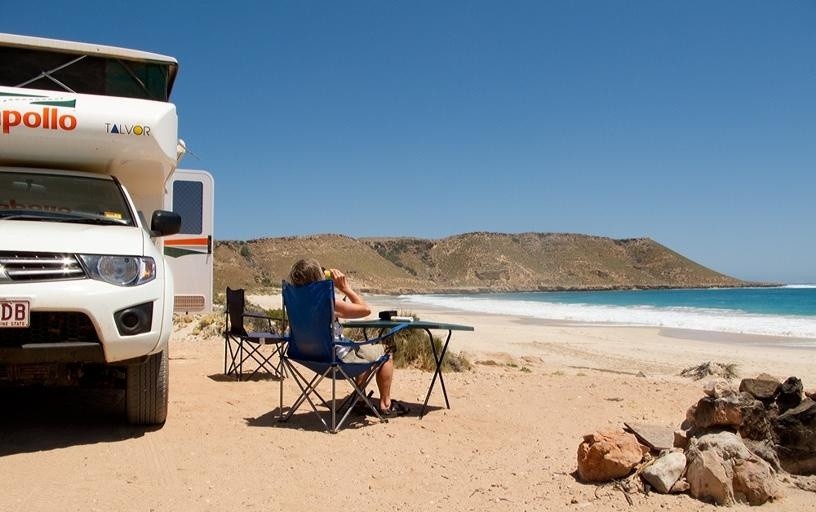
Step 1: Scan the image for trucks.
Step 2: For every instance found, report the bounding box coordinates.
[1,27,225,440]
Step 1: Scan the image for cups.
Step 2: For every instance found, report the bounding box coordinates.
[324,270,330,279]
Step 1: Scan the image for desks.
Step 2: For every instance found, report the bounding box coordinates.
[338,319,476,420]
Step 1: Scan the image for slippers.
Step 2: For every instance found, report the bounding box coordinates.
[353,400,379,414]
[378,400,411,418]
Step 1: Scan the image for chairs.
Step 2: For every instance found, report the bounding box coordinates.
[223,287,290,380]
[276,283,390,432]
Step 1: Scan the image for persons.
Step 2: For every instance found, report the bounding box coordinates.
[287,257,411,419]
